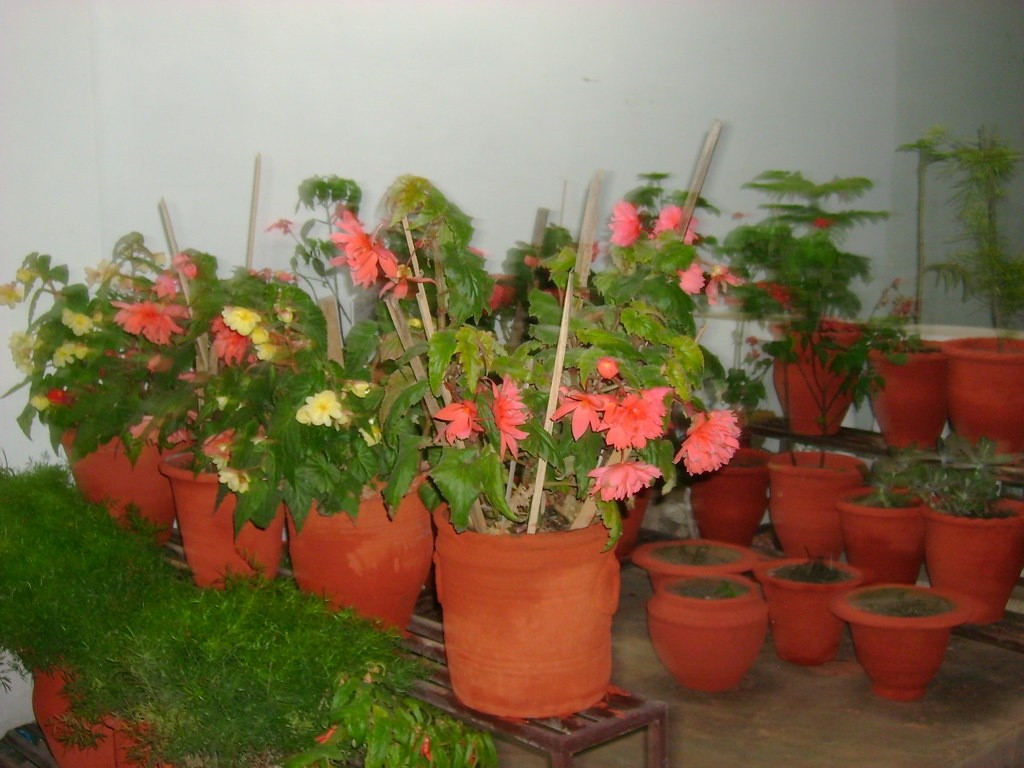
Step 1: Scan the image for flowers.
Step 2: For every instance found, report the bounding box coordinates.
[281,664,499,768]
[0,161,944,554]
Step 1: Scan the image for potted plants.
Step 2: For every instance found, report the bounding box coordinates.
[719,170,891,437]
[863,135,947,452]
[0,463,437,768]
[898,130,1024,464]
[835,444,935,586]
[633,535,958,701]
[913,433,1024,626]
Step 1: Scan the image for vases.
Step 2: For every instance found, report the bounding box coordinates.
[58,420,179,544]
[287,471,433,641]
[689,447,775,545]
[434,501,620,719]
[615,483,656,557]
[160,453,287,588]
[768,453,868,556]
